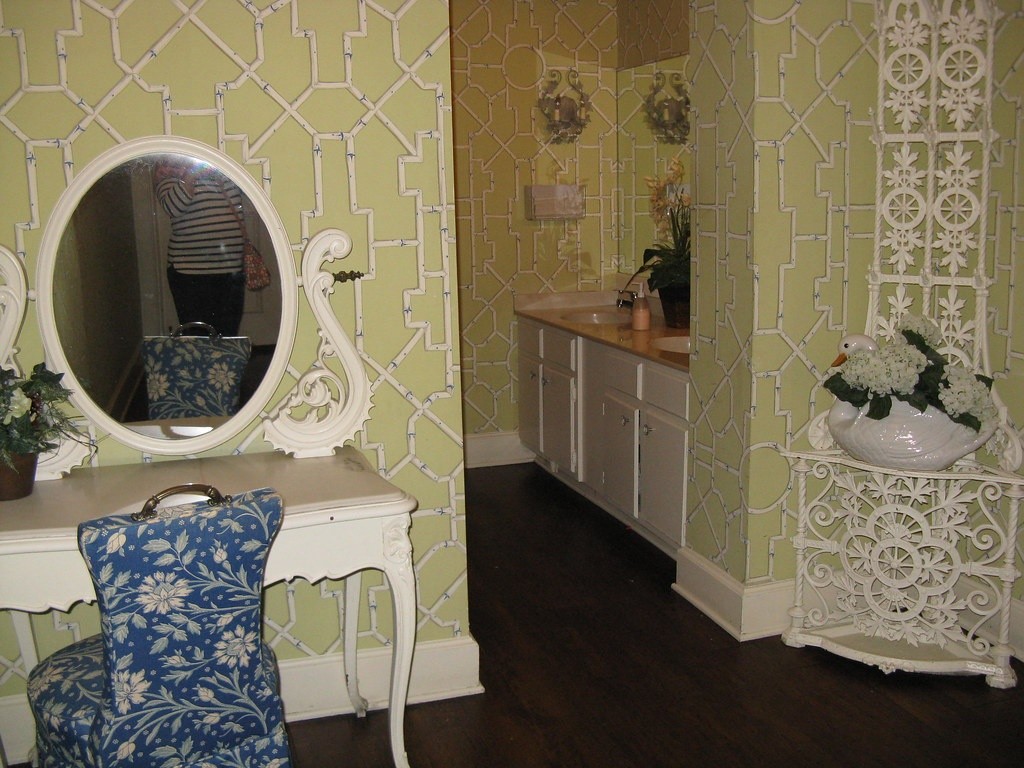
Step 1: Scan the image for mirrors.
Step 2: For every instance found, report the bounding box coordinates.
[37,136,299,456]
[617,53,696,277]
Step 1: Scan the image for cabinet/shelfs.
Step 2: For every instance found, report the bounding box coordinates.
[514,305,691,561]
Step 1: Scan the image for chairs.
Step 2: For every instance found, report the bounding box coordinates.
[142,322,250,418]
[27,483,291,768]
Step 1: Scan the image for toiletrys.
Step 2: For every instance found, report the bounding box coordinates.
[631,281,650,331]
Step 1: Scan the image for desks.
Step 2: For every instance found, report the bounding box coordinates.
[0,444,419,768]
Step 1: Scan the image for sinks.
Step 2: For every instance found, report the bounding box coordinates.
[649,336,690,354]
[558,311,633,326]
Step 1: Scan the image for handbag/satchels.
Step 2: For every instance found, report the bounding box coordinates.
[243,242,271,291]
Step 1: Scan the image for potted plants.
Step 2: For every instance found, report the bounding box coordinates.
[0,362,98,501]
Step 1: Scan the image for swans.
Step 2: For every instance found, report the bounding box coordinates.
[827,333,999,473]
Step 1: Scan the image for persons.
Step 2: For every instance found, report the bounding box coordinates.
[153,156,247,338]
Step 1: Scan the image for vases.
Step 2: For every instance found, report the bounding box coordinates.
[658,285,691,329]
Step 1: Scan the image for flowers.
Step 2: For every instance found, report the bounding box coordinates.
[616,159,690,294]
[824,311,1000,433]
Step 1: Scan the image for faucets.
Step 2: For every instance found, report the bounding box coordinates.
[612,289,637,308]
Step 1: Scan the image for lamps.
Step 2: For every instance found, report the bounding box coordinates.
[643,71,691,144]
[540,67,593,143]
